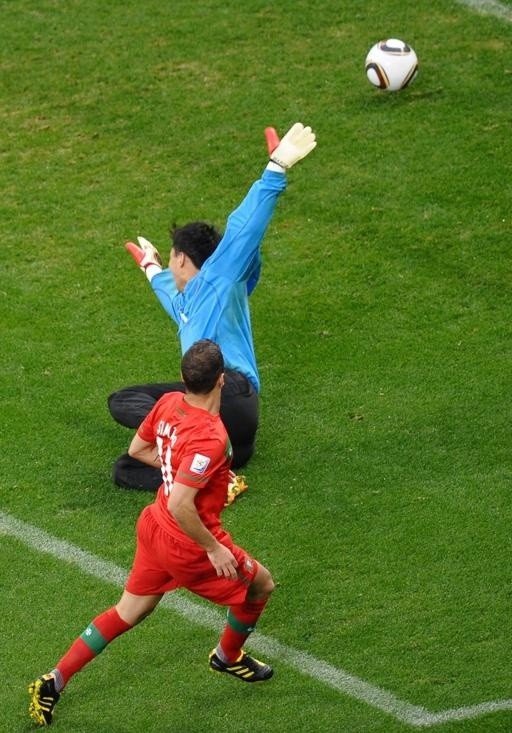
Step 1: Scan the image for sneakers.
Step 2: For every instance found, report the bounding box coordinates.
[27,672,60,728]
[208,647,274,683]
[222,469,249,508]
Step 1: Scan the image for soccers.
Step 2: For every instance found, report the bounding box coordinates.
[364,38,420,95]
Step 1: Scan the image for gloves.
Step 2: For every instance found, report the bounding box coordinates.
[263,123,318,173]
[124,236,163,282]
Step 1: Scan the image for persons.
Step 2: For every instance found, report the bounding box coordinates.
[25,335,279,728]
[102,118,321,511]
